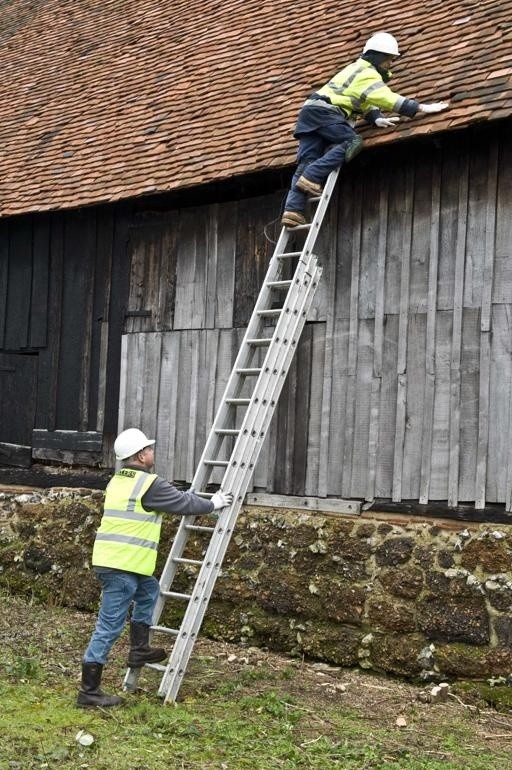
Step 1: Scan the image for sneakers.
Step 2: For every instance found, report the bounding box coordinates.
[295,175,323,197]
[281,211,309,237]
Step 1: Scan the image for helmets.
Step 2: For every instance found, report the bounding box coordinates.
[362,31,401,57]
[113,427,156,462]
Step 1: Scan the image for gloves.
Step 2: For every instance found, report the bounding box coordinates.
[375,116,400,128]
[420,100,449,114]
[209,488,235,510]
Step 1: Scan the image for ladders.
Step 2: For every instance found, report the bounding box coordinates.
[122,153,341,706]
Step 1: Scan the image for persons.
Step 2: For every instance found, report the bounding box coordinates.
[280,31,450,230]
[71,427,234,711]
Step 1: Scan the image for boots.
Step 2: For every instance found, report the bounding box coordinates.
[127,619,168,668]
[75,661,126,709]
